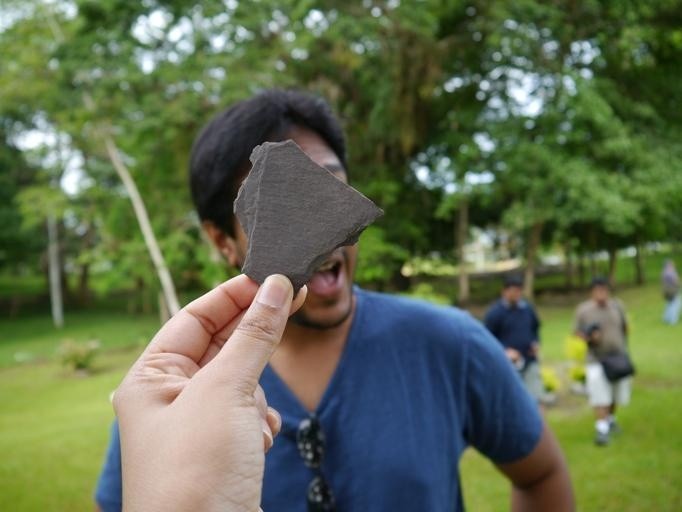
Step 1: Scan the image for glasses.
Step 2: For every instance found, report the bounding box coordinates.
[283,412,336,511]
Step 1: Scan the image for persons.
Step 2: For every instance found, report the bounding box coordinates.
[480,272,545,409]
[568,276,633,447]
[93,86,577,511]
[658,257,681,326]
[111,271,310,511]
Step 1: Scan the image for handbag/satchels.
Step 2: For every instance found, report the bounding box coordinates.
[601,350,637,382]
